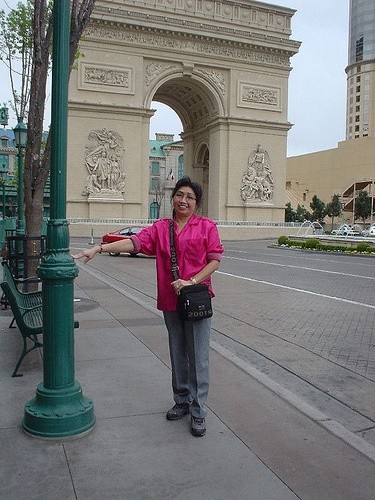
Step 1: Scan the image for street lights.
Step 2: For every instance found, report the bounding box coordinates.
[12,117,28,277]
[0,163,9,219]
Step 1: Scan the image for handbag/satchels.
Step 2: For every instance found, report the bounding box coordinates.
[177,284,213,321]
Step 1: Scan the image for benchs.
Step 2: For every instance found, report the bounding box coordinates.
[0,257,79,377]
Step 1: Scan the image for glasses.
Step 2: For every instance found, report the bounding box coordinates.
[175,193,197,201]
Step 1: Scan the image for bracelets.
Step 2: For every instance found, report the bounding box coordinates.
[190,276,197,285]
[99,244,103,254]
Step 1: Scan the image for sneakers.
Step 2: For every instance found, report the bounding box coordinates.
[190,415,206,436]
[166,403,190,420]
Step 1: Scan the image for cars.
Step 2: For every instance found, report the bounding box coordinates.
[330,225,359,235]
[100,225,148,257]
[361,225,375,238]
[312,223,325,234]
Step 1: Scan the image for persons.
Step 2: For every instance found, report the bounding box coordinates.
[72,177,224,435]
[86,132,119,158]
[86,151,121,189]
[243,145,273,198]
